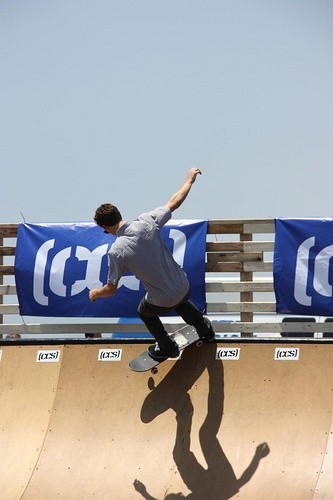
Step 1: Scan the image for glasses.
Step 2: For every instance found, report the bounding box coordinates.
[103,230,109,234]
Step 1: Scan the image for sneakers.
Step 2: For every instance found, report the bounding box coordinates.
[203,317,216,341]
[146,344,179,358]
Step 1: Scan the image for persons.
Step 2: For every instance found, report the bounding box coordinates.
[87,167,215,359]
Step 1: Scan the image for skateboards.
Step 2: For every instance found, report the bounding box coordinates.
[128,317,211,374]
